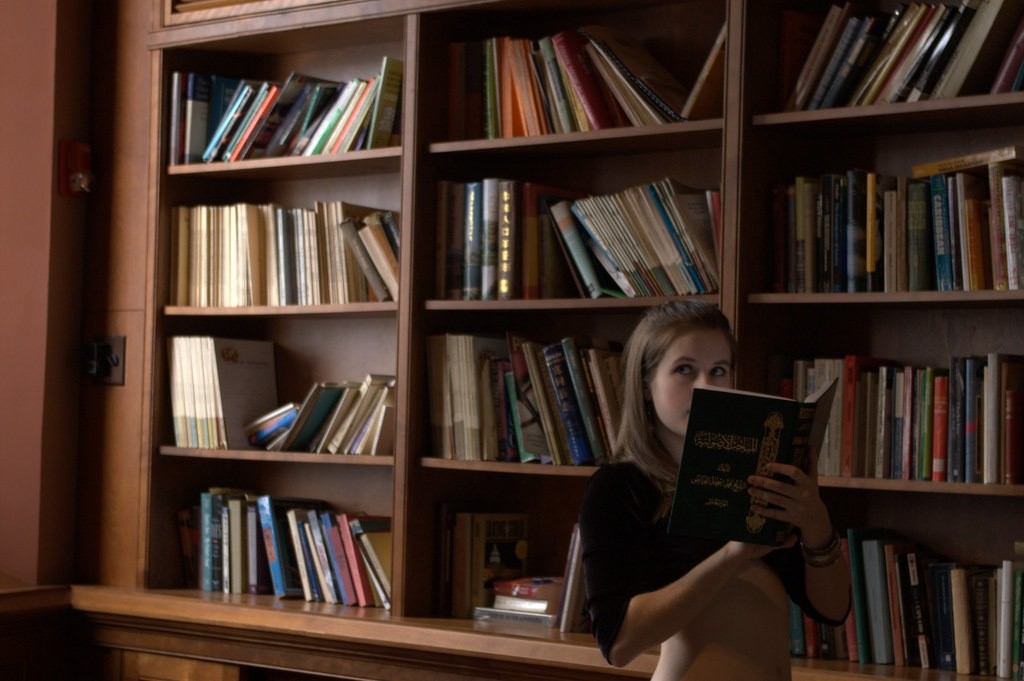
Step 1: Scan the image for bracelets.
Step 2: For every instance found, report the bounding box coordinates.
[801,528,844,569]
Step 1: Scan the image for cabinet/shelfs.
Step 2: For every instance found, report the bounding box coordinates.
[133,0,1024,681]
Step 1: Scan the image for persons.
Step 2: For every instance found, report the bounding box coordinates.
[578,298,853,681]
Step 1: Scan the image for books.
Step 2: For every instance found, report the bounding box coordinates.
[779,351,1024,484]
[481,21,727,141]
[430,336,631,466]
[784,1,1024,109]
[174,332,395,463]
[786,147,1024,294]
[169,56,405,165]
[174,198,407,307]
[174,475,390,612]
[784,525,1023,680]
[437,177,724,299]
[664,375,840,546]
[436,504,590,640]
